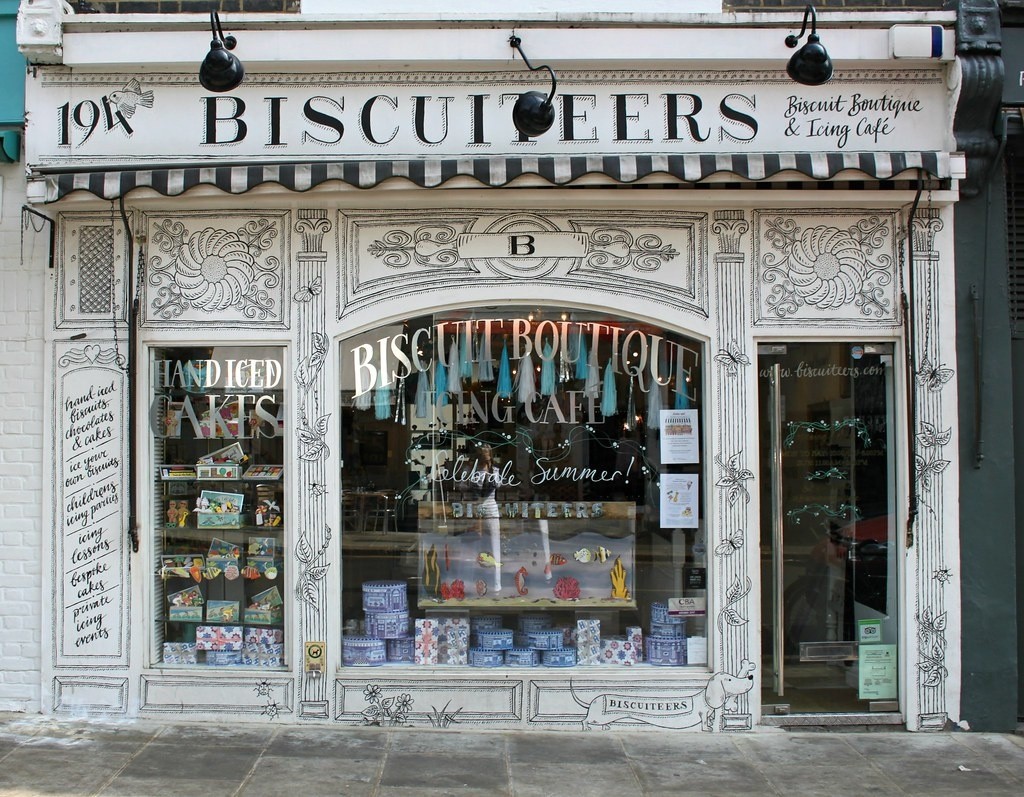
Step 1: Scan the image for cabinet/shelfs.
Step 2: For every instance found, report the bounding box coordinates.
[153,478,286,629]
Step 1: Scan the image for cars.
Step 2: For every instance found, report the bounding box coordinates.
[807,515,888,611]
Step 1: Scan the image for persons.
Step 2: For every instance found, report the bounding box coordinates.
[511,437,555,582]
[454,441,503,597]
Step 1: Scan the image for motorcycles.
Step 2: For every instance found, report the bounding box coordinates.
[784,530,887,656]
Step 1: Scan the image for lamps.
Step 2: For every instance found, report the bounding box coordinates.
[785,3,835,86]
[509,38,557,136]
[199,9,246,93]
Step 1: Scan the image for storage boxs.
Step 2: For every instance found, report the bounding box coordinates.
[577,618,599,664]
[414,617,468,665]
[244,627,284,643]
[162,641,197,663]
[242,644,284,665]
[206,650,240,665]
[196,625,243,650]
